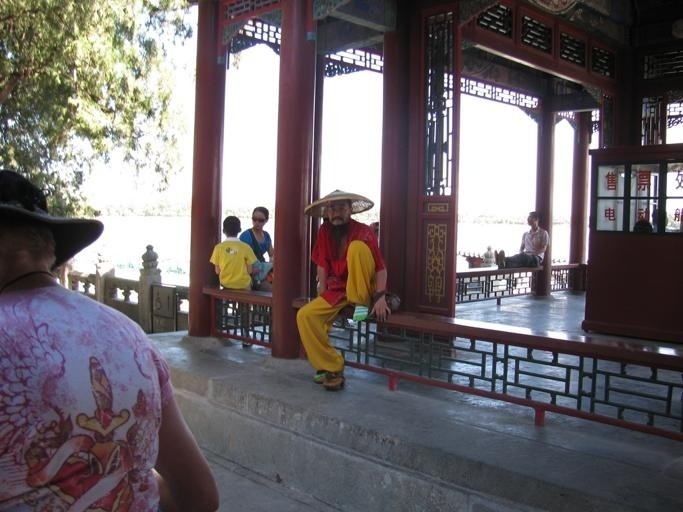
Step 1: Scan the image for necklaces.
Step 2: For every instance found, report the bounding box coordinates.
[0,270,54,293]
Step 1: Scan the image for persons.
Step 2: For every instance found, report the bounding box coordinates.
[482,246,494,260]
[294,189,390,391]
[633,219,653,233]
[208,215,257,347]
[0,170,220,512]
[369,222,379,242]
[494,212,549,269]
[651,209,668,233]
[238,207,273,291]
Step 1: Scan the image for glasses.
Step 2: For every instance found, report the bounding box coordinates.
[251,217,268,223]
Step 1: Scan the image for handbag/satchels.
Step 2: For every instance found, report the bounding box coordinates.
[373,291,402,317]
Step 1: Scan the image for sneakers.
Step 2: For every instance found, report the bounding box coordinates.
[498,251,506,268]
[495,250,500,265]
[312,367,346,388]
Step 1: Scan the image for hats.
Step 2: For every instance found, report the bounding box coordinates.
[1,170,104,271]
[305,189,374,219]
